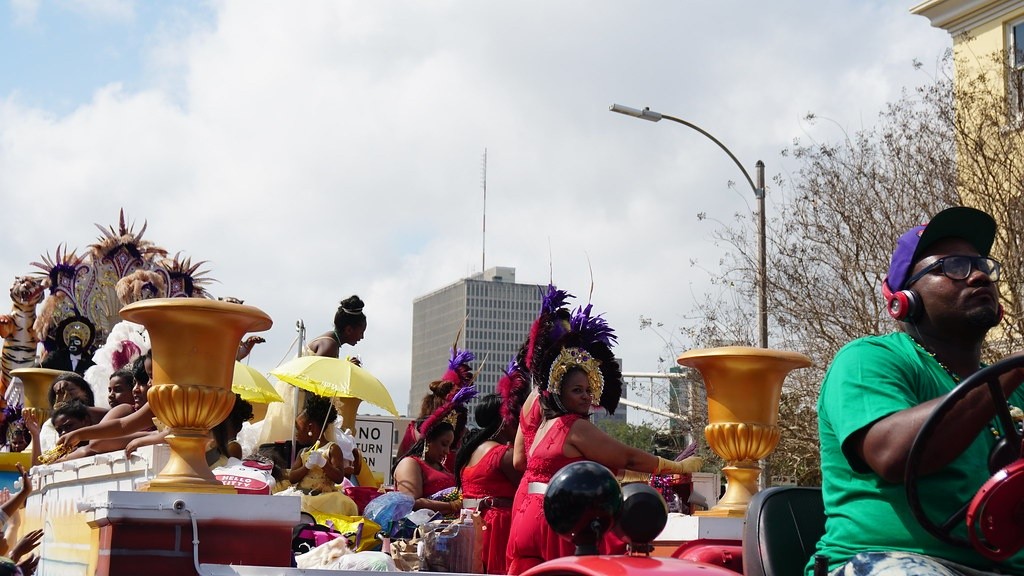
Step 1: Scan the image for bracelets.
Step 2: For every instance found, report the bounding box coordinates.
[8,551,19,563]
[449,501,455,513]
[21,492,27,499]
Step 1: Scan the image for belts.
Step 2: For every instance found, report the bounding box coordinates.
[527,482,549,495]
[462,496,513,513]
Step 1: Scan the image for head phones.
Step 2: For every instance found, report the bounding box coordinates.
[881,275,1004,326]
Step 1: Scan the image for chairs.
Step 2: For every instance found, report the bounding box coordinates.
[741,484,828,576]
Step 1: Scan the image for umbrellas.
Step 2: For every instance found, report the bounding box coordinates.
[231,360,283,406]
[267,357,399,451]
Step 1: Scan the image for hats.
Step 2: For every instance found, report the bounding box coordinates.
[886,206,998,295]
[417,285,622,435]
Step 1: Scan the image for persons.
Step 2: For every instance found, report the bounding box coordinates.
[803,206,1024,576]
[0,291,707,576]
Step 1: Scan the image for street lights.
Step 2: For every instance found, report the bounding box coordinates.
[608,100,777,498]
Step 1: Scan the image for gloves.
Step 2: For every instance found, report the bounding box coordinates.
[656,455,704,476]
[305,451,323,470]
[308,452,326,468]
[619,469,653,483]
[449,498,464,514]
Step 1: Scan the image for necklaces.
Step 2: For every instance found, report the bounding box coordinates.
[334,328,343,347]
[38,443,72,464]
[908,334,1024,438]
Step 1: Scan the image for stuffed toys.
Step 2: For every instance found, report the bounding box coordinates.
[236,335,266,361]
[0,274,48,424]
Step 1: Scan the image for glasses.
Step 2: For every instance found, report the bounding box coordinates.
[901,254,1003,289]
